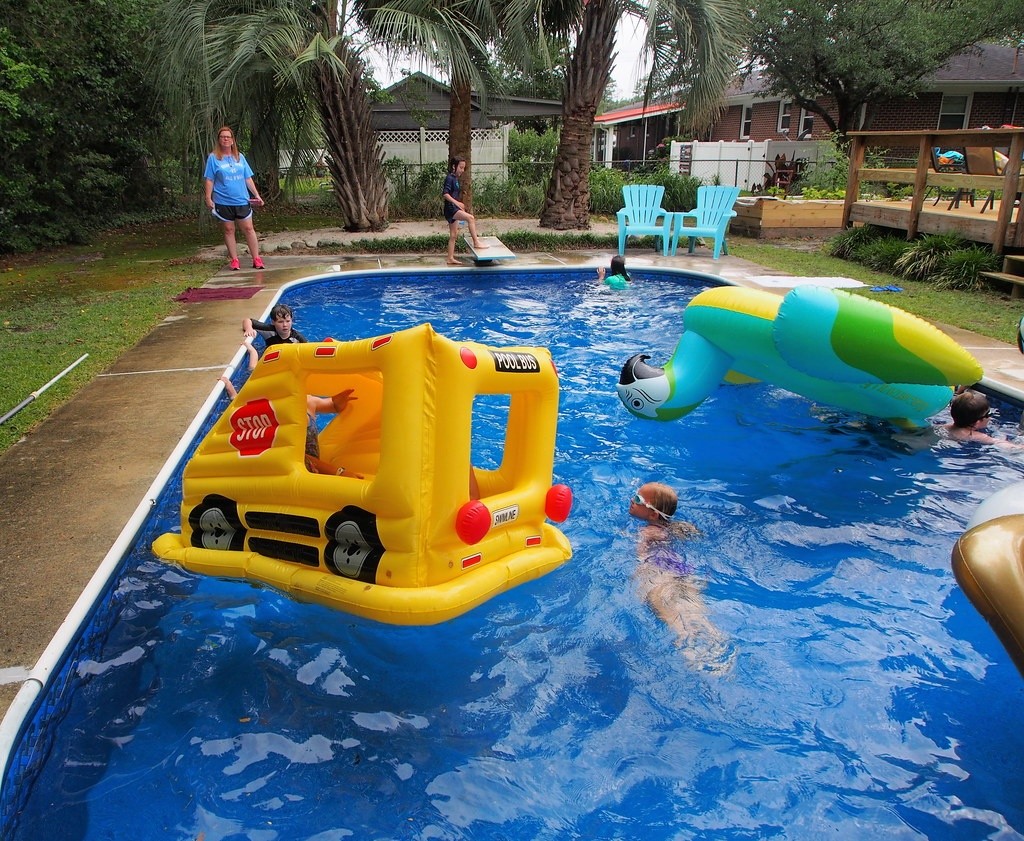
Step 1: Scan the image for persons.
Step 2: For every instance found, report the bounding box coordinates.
[442,156,490,265]
[204,126,265,271]
[593,256,639,290]
[943,383,1023,452]
[625,480,687,527]
[215,304,365,490]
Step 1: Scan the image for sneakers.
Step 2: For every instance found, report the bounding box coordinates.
[253,256,265,269]
[231,257,241,270]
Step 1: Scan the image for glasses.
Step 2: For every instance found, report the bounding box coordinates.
[986,410,992,418]
[633,492,645,506]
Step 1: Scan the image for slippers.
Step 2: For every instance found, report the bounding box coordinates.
[885,284,904,292]
[870,286,889,291]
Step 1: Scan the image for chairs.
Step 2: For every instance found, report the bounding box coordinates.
[616,185,673,256]
[670,185,742,259]
[923,147,975,210]
[947,146,1009,213]
